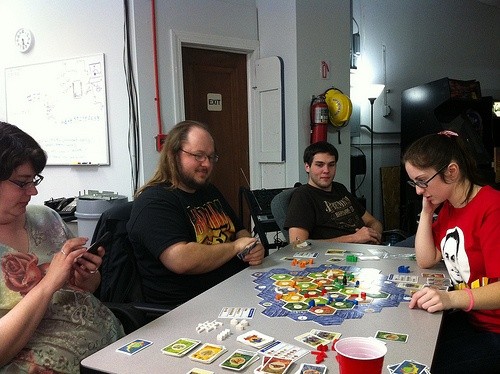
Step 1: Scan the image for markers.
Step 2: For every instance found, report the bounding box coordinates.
[78,162,91,164]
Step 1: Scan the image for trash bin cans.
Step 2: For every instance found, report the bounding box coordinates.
[74,189,128,248]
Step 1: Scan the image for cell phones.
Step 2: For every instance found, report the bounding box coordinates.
[76,232,110,266]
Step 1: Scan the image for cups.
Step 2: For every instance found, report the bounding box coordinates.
[334,337,388,374]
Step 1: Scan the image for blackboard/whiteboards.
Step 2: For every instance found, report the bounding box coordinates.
[5,52,110,166]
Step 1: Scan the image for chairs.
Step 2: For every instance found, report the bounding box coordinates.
[237,183,301,256]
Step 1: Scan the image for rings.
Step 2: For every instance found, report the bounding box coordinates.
[90,268,98,273]
[60,250,68,255]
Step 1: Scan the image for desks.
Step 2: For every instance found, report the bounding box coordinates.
[80,239,450,374]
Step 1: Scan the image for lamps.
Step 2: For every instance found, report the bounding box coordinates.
[364,83,385,216]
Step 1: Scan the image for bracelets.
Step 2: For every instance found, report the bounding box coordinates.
[463,288,473,312]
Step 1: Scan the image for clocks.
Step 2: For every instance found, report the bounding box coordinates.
[16,28,34,53]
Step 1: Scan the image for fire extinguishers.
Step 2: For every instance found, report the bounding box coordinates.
[310,94,329,143]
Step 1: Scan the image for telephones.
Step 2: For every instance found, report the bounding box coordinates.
[56,197,76,217]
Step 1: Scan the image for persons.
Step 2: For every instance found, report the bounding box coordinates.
[127,120,264,310]
[0,121,105,366]
[404,131,500,374]
[285,142,415,248]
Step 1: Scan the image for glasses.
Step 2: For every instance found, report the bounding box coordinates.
[406,164,448,189]
[178,148,219,164]
[7,174,44,190]
